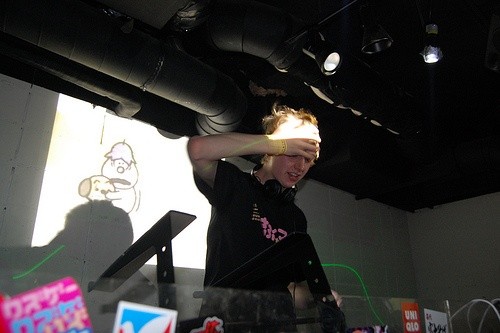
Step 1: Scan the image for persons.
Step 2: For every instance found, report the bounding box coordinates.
[187,104,343,333]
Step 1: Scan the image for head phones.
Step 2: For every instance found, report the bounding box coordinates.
[250,162,298,206]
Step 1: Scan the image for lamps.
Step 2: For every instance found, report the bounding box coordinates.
[302,35,344,75]
[419,23,443,63]
[360,3,394,54]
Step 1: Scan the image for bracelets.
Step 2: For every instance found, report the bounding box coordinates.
[278,135,289,154]
